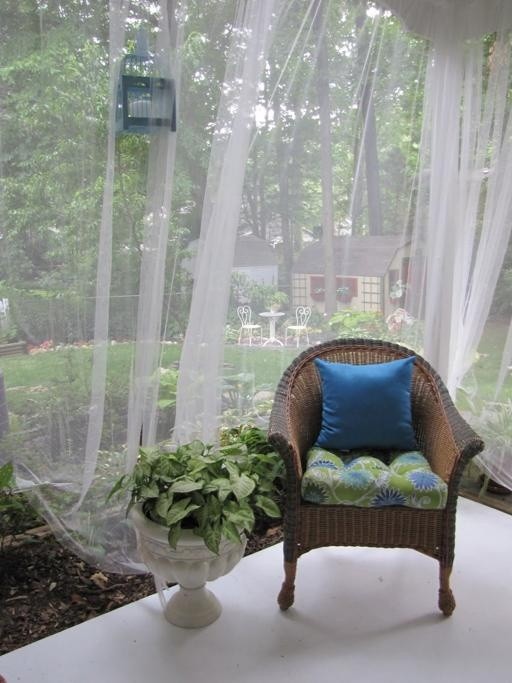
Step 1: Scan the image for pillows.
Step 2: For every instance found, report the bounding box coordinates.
[313,349,422,457]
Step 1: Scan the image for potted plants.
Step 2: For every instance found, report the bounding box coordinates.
[103,441,282,632]
[309,288,327,301]
[335,286,353,303]
[263,292,289,313]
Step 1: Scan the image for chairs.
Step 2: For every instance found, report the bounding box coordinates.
[237,303,264,348]
[268,342,486,621]
[285,306,312,347]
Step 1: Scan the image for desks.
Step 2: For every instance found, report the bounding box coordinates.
[258,312,287,347]
